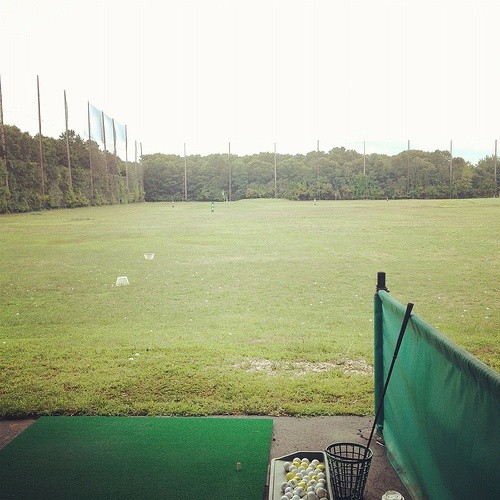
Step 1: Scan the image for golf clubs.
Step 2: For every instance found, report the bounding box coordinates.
[349,303,414,494]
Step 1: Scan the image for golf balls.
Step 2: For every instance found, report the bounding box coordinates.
[281,457,327,500]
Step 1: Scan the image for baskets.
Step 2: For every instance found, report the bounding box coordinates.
[325,441,374,500]
